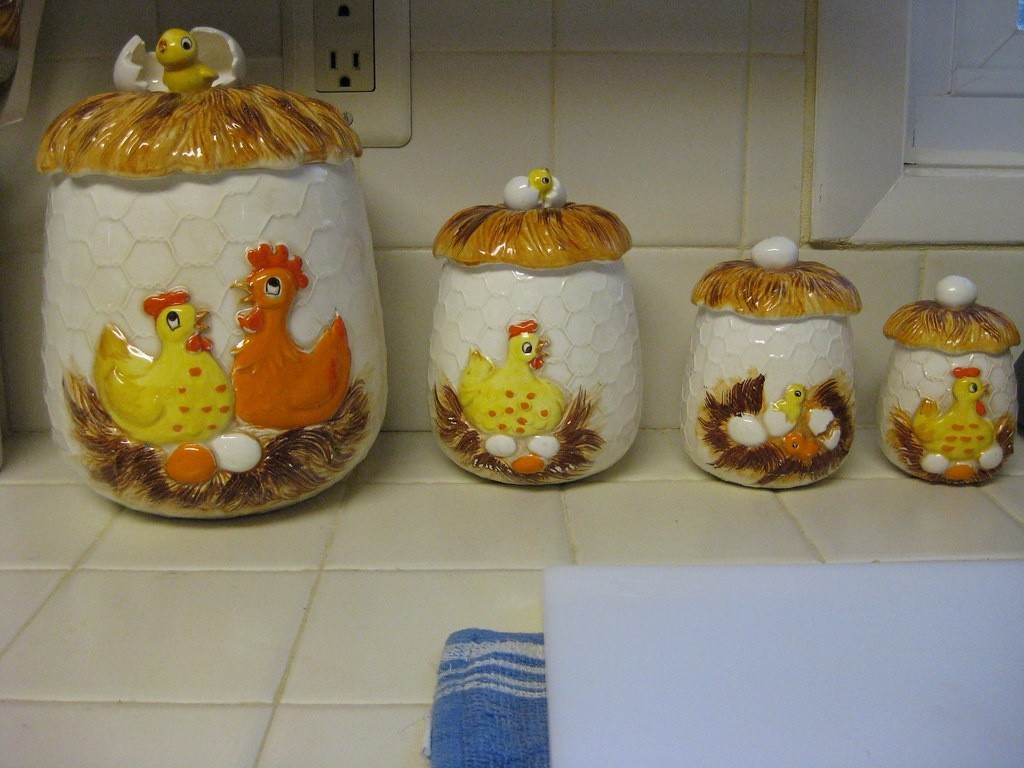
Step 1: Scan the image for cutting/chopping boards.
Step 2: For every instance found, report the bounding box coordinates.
[540,562,1024,768]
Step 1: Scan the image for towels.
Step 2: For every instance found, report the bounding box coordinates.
[424,627,550,768]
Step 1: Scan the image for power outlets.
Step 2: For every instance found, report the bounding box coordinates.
[279,0,412,148]
